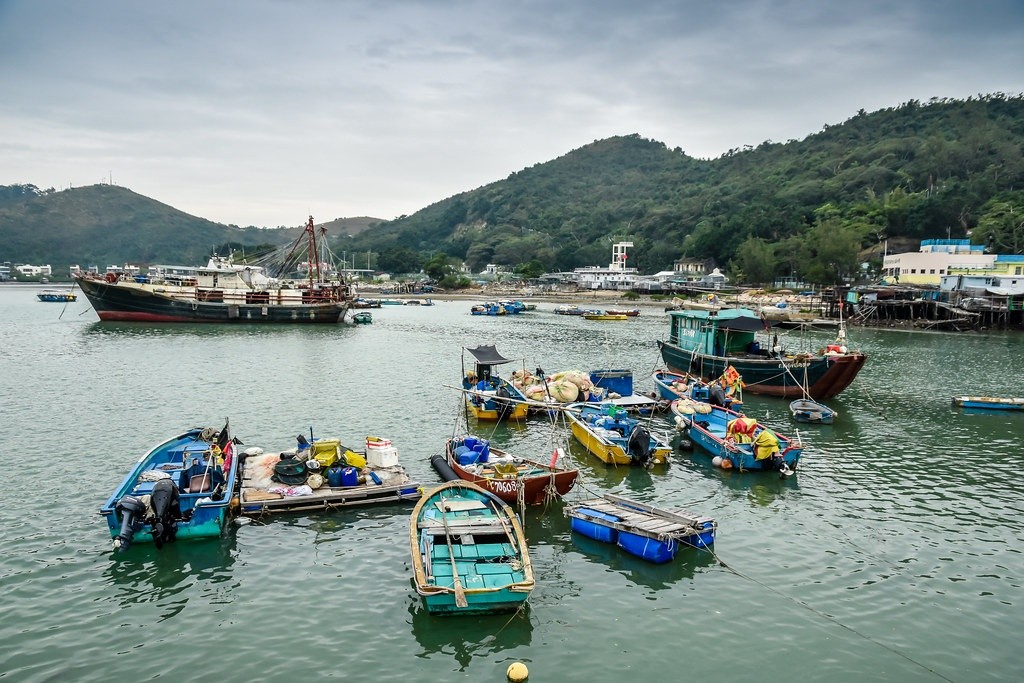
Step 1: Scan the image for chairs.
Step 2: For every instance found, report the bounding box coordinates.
[183,473,210,493]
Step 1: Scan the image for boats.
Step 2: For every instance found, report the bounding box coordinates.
[235,426,425,517]
[470,299,538,314]
[445,432,579,505]
[36,289,77,303]
[562,492,717,565]
[553,304,641,320]
[408,478,536,617]
[99,425,239,556]
[655,287,869,401]
[72,215,357,325]
[354,296,436,308]
[951,396,1024,410]
[459,344,529,420]
[653,369,803,479]
[505,368,673,466]
[789,397,838,424]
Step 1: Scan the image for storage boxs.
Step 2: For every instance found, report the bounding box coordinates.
[589,369,633,396]
[494,463,519,479]
[366,438,393,448]
[343,450,366,469]
[366,447,399,467]
[314,437,343,453]
[315,451,336,467]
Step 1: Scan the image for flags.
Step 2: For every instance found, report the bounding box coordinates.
[760,312,770,332]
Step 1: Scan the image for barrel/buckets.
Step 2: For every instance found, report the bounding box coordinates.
[329,468,341,486]
[460,451,479,465]
[473,445,488,462]
[464,437,477,452]
[341,467,357,486]
[477,440,490,455]
[453,447,470,463]
